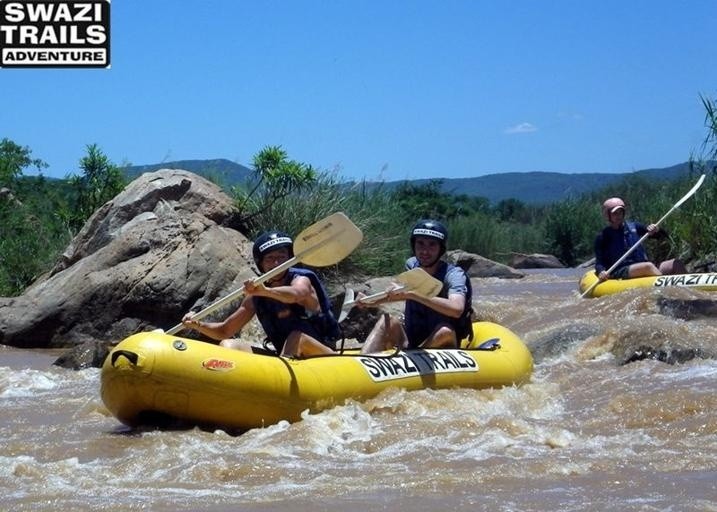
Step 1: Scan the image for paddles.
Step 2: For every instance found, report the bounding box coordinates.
[341,267,444,310]
[165,211,363,335]
[580,173,705,296]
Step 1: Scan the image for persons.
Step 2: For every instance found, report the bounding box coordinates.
[594,196,689,283]
[355,218,474,355]
[180,230,346,363]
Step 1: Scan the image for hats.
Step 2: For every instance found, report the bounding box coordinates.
[610,205,625,213]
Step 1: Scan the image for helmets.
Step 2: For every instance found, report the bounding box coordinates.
[603,197,625,220]
[253,233,293,273]
[410,220,448,256]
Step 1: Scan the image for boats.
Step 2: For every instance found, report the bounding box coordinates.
[98,317,535,438]
[576,267,716,297]
